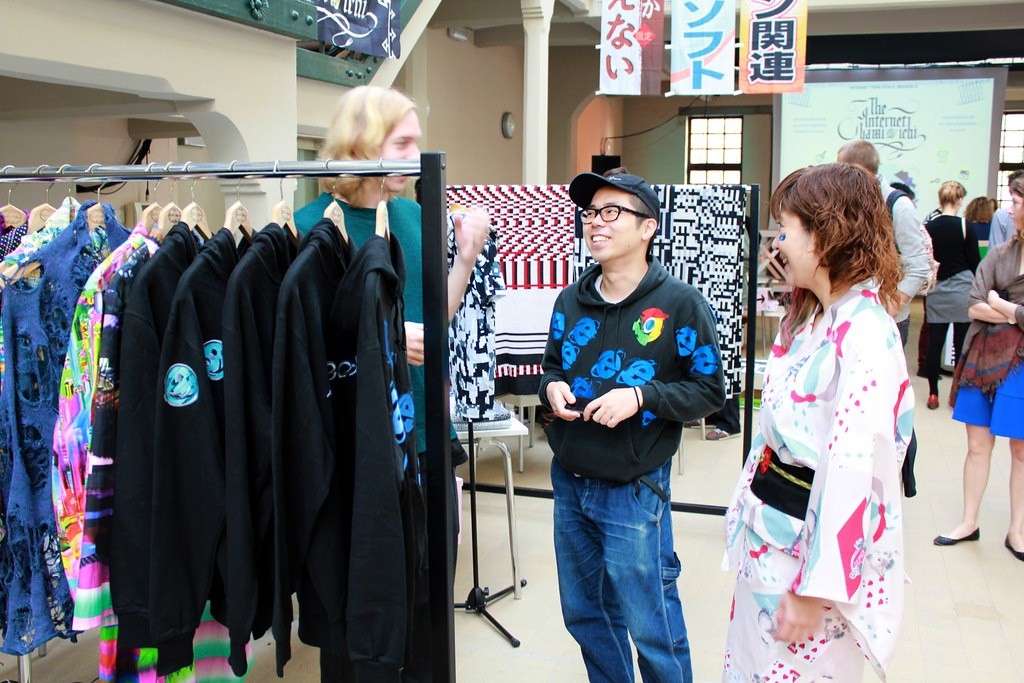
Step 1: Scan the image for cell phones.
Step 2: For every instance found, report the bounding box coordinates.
[565,397,601,415]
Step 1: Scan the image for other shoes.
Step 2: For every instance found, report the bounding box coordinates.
[934,527,981,544]
[1005,536,1024,561]
[927,395,939,409]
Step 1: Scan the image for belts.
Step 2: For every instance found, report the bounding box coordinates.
[639,475,667,503]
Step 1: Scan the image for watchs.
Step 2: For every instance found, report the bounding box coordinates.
[1007,317,1015,324]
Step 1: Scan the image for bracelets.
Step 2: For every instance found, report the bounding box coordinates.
[632,386,641,414]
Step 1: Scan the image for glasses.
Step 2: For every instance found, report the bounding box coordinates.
[578,205,649,224]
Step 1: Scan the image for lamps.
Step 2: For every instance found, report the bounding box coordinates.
[502,113,516,138]
[448,26,468,42]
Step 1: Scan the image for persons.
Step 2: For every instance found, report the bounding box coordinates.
[536,172,725,683]
[286,85,490,519]
[683,393,741,441]
[838,141,940,353]
[721,161,916,683]
[933,175,1024,560]
[916,167,1024,413]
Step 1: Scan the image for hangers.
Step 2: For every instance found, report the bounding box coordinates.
[1,158,389,241]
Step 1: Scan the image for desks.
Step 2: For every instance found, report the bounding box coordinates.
[500,395,542,473]
[452,415,528,599]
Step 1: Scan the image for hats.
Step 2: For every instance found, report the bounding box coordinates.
[568,172,660,226]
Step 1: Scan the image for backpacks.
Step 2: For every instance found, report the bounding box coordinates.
[885,189,941,296]
